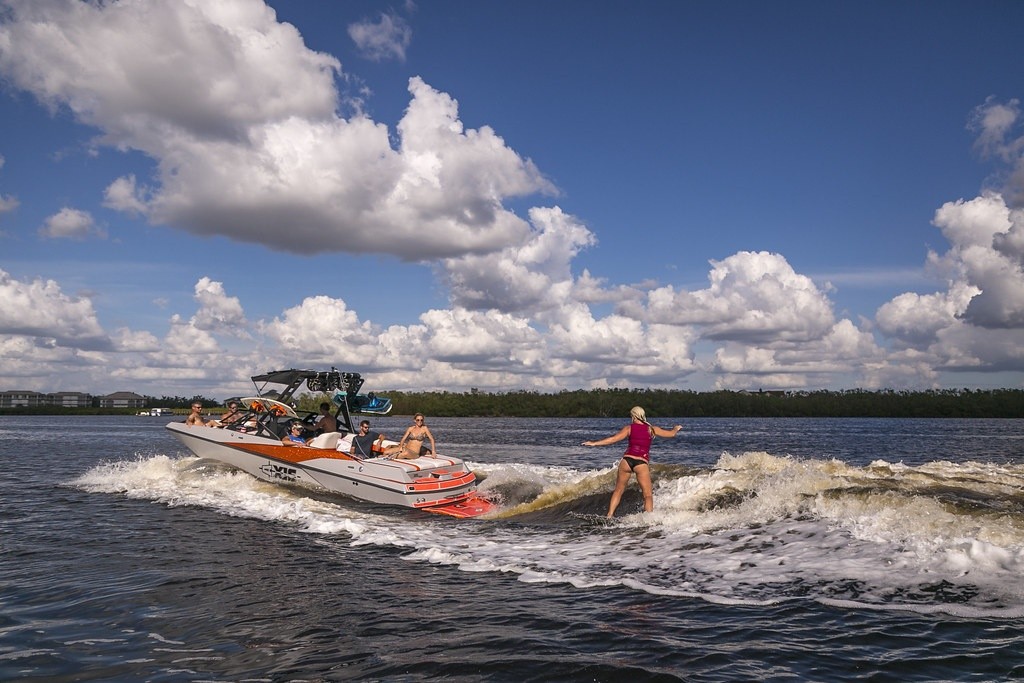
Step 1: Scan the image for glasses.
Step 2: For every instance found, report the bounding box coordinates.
[415,419,424,422]
[297,428,303,431]
[319,409,321,410]
[231,406,237,409]
[362,426,369,429]
[196,407,202,409]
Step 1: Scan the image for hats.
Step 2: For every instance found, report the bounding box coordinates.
[292,421,304,430]
[631,406,645,420]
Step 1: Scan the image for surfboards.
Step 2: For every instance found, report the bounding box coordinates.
[239,397,299,419]
[572,512,621,526]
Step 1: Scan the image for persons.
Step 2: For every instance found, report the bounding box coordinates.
[580,406,683,520]
[383,413,437,459]
[186,400,221,427]
[282,423,326,448]
[294,402,336,446]
[221,402,244,425]
[350,420,385,459]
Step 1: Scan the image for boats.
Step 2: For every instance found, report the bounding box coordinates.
[150,407,173,417]
[136,412,149,417]
[164,368,497,518]
